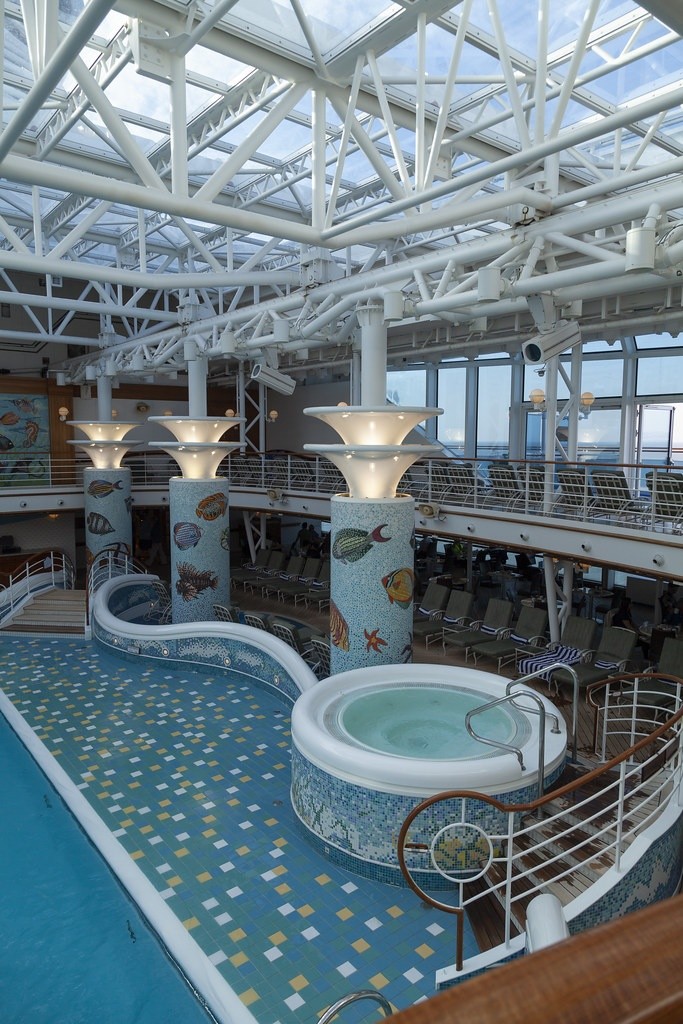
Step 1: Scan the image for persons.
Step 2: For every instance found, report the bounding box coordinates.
[612,598,641,636]
[662,582,677,612]
[293,522,331,556]
[1,534,22,553]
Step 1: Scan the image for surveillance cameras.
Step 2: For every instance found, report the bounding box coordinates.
[250,364,296,397]
[520,320,582,365]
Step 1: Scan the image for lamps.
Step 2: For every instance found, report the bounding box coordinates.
[577,393,595,422]
[529,389,547,412]
[268,410,279,423]
[224,408,239,418]
[111,409,118,420]
[163,409,173,416]
[58,406,68,422]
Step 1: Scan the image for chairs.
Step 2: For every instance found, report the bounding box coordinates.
[412,540,683,733]
[212,544,332,677]
[224,450,350,494]
[399,461,683,534]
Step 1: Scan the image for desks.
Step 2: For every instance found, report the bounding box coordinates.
[0,547,62,590]
